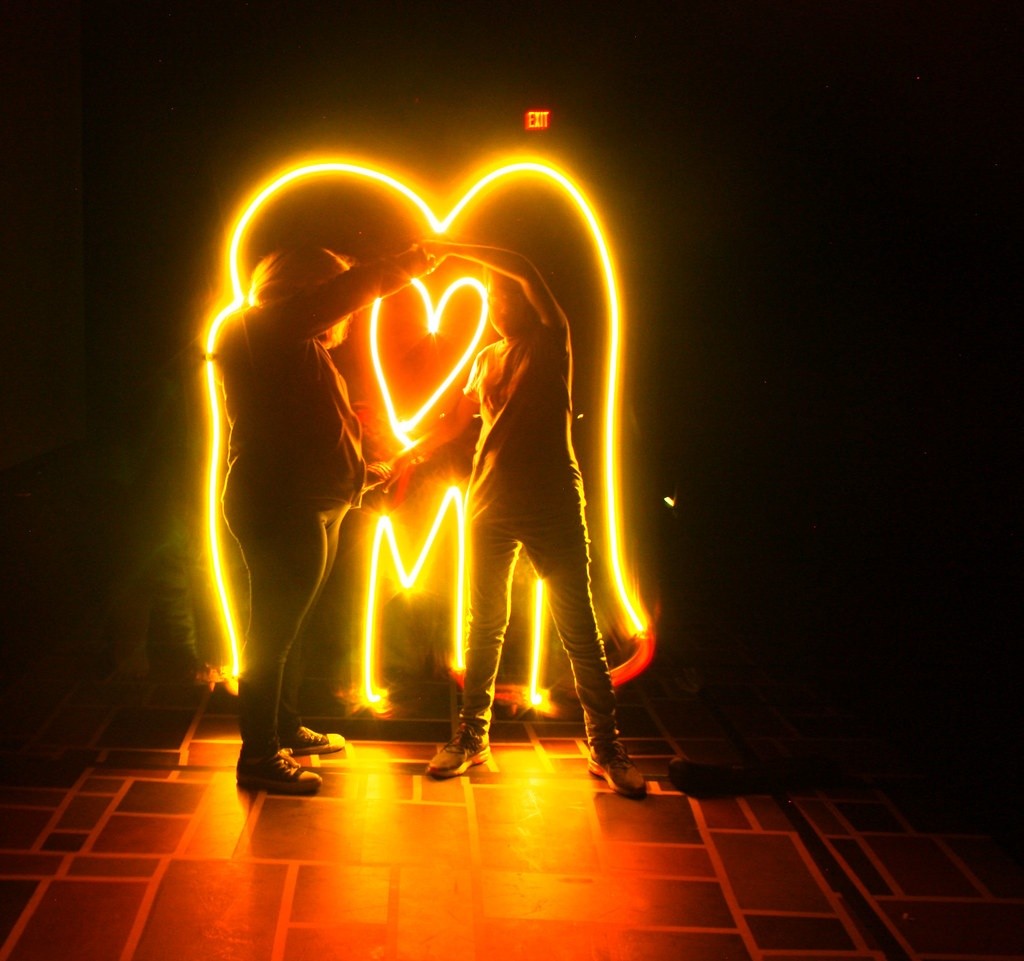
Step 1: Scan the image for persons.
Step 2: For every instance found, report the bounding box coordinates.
[207,238,452,793]
[381,236,653,801]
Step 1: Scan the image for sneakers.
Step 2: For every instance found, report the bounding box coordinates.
[278,724,346,754]
[234,753,321,792]
[427,724,491,781]
[587,739,647,798]
